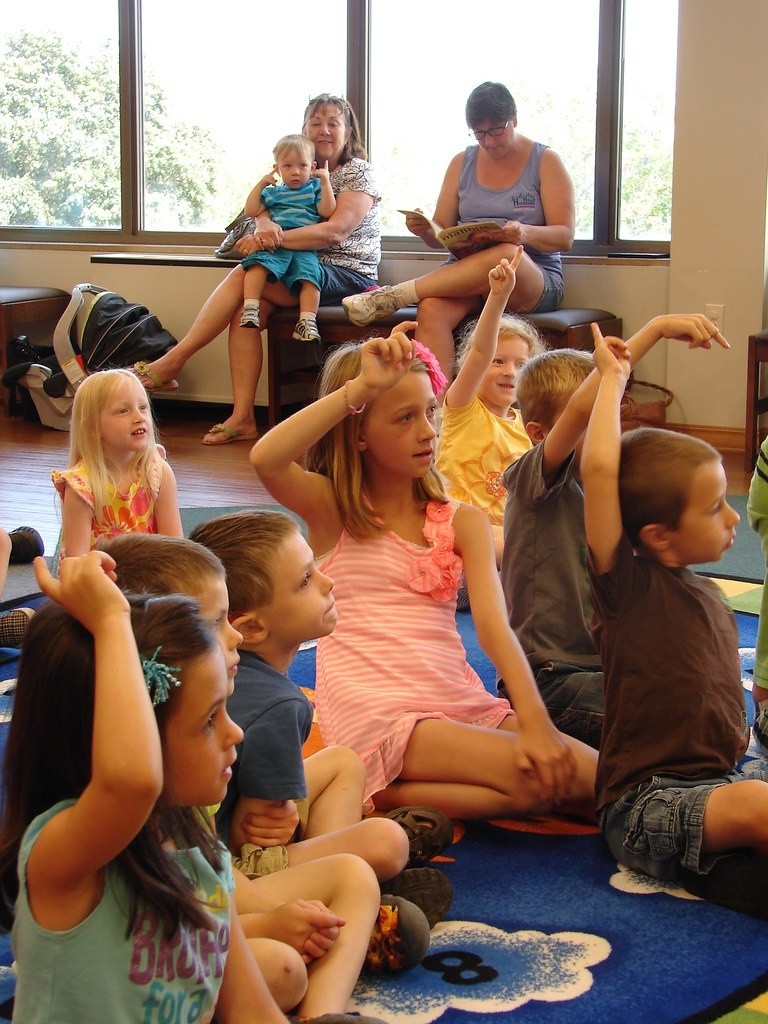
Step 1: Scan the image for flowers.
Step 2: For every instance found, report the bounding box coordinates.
[411,339,449,396]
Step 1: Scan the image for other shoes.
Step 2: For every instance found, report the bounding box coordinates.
[0,607,36,649]
[362,891,430,975]
[9,526,45,567]
[291,1012,387,1024]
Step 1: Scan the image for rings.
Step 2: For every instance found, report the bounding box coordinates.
[244,249,248,253]
[260,240,266,242]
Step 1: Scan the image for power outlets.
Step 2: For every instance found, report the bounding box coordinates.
[704,304,724,337]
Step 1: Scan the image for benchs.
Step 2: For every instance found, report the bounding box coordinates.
[265,304,625,429]
[0,284,77,418]
[744,325,768,473]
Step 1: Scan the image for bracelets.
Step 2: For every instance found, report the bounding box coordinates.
[344,380,367,415]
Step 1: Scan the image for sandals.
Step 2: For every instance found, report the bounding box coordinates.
[384,805,454,864]
[381,866,452,931]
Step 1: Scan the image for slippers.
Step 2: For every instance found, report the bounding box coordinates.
[200,423,259,444]
[125,362,179,391]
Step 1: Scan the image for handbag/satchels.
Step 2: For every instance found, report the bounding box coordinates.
[620,378,673,434]
[215,205,257,259]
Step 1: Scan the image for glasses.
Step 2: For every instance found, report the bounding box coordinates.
[468,118,513,141]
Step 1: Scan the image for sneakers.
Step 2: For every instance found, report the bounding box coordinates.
[341,285,404,327]
[239,303,260,328]
[292,318,320,344]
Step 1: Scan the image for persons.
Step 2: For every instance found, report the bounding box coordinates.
[239,135,337,343]
[128,93,382,444]
[250,321,600,820]
[342,82,576,437]
[0,509,453,1024]
[581,324,768,883]
[501,313,730,764]
[429,244,558,614]
[58,370,185,576]
